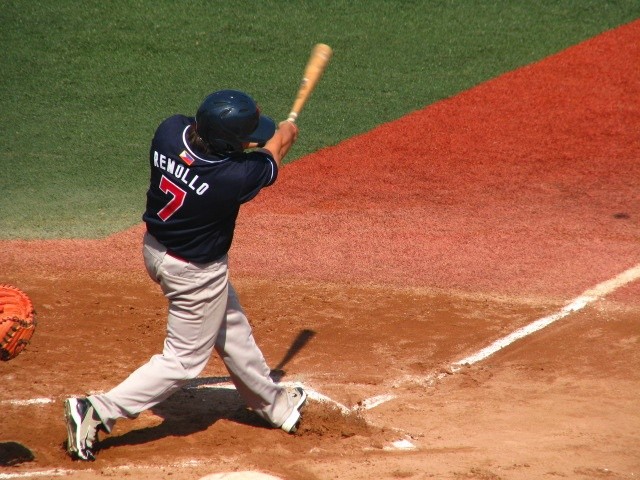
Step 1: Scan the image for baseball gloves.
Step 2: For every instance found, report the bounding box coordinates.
[1,284,35,360]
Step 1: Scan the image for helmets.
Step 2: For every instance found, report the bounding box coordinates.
[196,90,276,156]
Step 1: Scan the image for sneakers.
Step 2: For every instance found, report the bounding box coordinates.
[64,398,103,461]
[279,387,306,433]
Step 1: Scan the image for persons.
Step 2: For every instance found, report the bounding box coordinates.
[62,90,307,461]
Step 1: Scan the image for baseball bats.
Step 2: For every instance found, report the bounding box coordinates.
[288,43,332,120]
